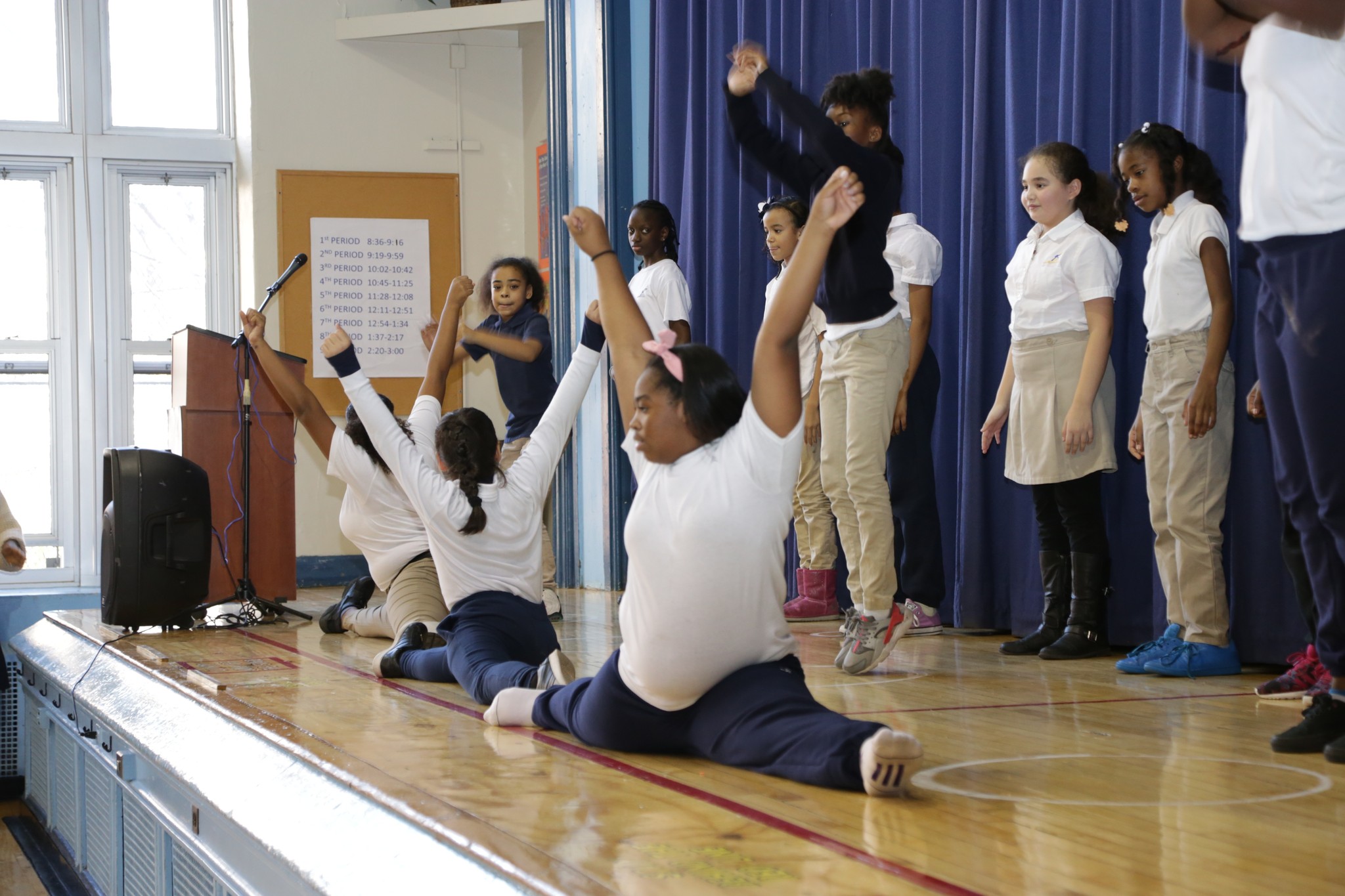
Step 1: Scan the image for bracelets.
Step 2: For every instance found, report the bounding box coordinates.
[590,250,614,262]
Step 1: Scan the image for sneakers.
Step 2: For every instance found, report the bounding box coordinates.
[899,598,943,637]
[535,650,576,690]
[1115,623,1184,673]
[320,575,375,635]
[1256,643,1327,700]
[1144,637,1241,677]
[373,621,428,678]
[842,600,913,675]
[1323,739,1345,766]
[1302,671,1334,707]
[1270,693,1345,754]
[835,605,860,669]
[418,632,448,651]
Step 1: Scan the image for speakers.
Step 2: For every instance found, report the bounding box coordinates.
[101,448,211,632]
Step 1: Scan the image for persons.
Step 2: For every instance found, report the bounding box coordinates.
[1184,0,1345,762]
[0,494,27,572]
[420,256,562,618]
[238,276,476,651]
[1114,122,1239,676]
[978,144,1122,658]
[628,200,693,347]
[319,301,604,705]
[883,206,944,637]
[755,194,841,622]
[485,166,921,796]
[725,44,912,676]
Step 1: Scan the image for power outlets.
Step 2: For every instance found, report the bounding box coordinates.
[192,806,199,835]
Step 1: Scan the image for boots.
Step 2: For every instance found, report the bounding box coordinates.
[782,566,804,609]
[1000,549,1072,657]
[783,567,840,620]
[1038,551,1108,659]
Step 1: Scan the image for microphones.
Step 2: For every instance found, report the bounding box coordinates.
[266,254,307,295]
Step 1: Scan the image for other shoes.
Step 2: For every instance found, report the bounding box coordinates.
[542,589,565,622]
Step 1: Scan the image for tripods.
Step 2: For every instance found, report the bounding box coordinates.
[192,293,315,624]
